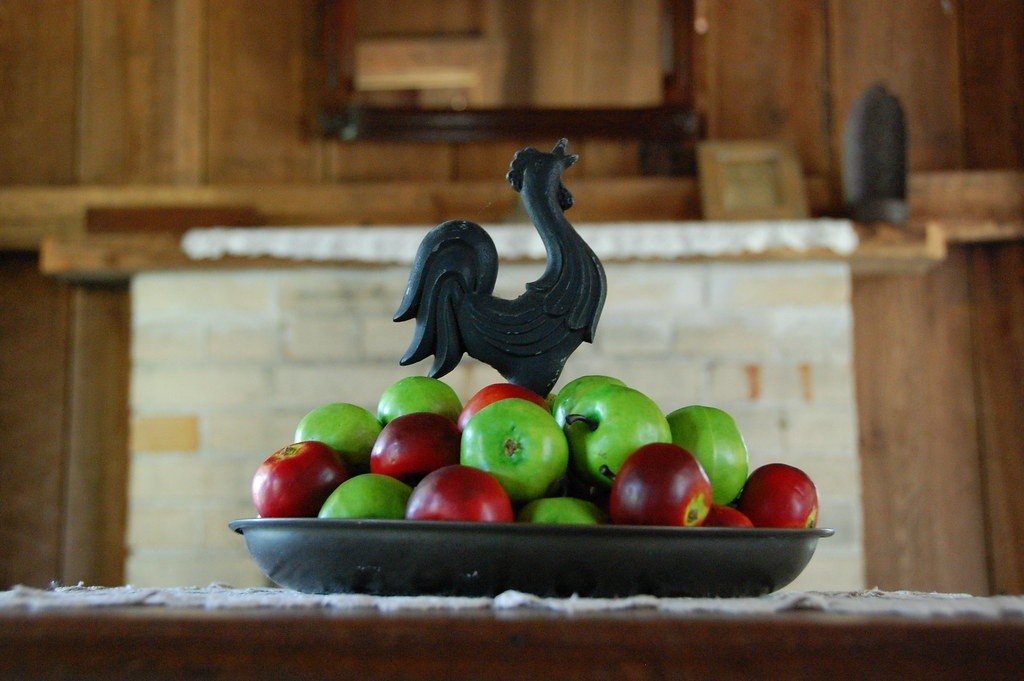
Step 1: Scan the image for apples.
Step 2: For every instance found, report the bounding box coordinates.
[252,373,820,528]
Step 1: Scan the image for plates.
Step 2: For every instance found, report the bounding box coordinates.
[228,515,836,599]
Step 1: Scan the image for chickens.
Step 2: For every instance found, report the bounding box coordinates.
[392,135,607,401]
